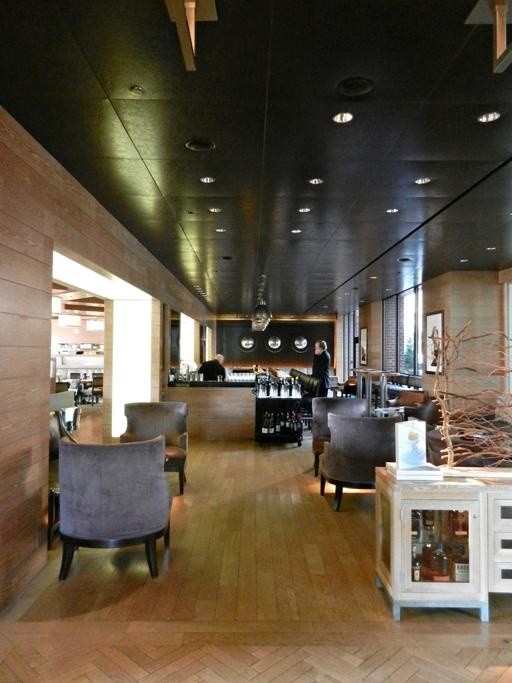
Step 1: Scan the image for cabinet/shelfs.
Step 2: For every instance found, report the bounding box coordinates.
[373,466,512,623]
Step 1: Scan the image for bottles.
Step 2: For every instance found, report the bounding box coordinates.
[261,410,303,435]
[412,508,469,582]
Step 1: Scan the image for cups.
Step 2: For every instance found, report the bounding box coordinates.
[177,371,203,382]
[218,375,222,382]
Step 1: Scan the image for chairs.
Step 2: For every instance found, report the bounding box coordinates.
[386,390,449,433]
[371,373,424,399]
[311,398,402,512]
[55,373,103,406]
[57,401,188,584]
[287,368,321,431]
[338,376,365,399]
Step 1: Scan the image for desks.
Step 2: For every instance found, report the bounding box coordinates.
[350,368,400,407]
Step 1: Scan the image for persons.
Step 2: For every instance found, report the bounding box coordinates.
[198,354,226,382]
[308,340,330,398]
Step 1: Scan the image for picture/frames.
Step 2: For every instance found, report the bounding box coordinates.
[360,327,368,366]
[425,309,445,376]
